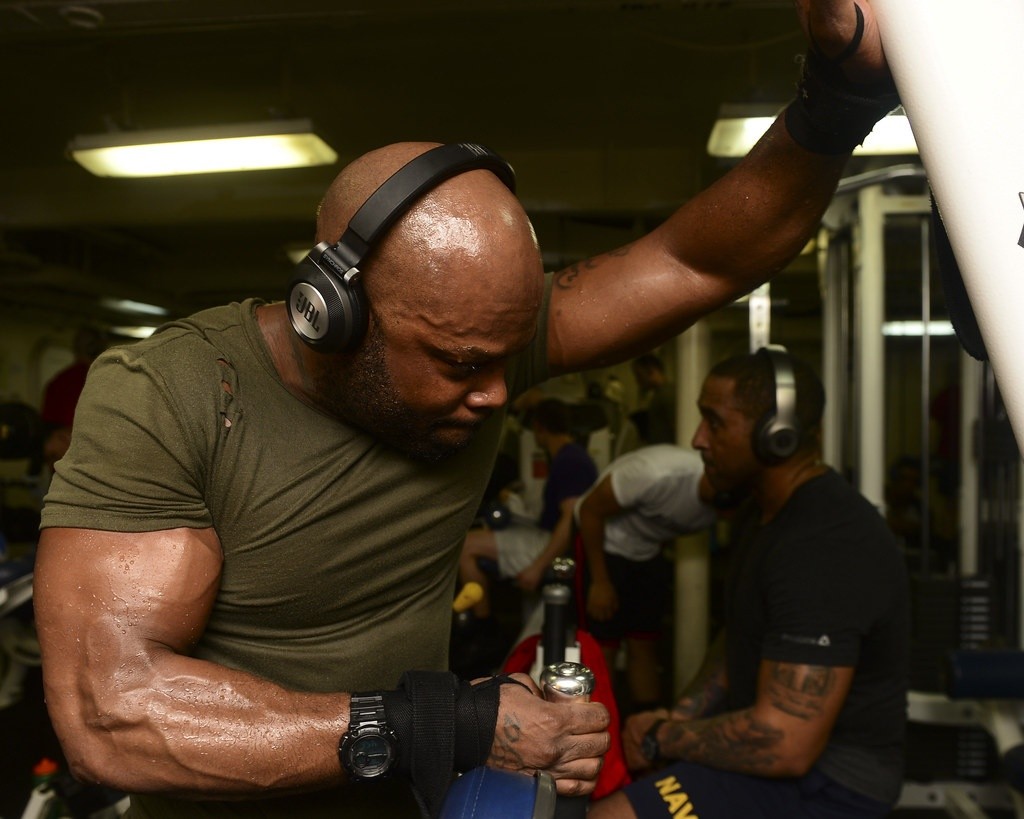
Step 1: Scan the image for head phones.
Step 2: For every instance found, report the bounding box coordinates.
[284,142,516,355]
[752,343,801,467]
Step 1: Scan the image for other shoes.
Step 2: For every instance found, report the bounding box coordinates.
[454,615,500,655]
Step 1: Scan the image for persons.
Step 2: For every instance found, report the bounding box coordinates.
[0,0,918,819]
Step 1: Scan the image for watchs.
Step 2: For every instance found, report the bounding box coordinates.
[339,690,399,783]
